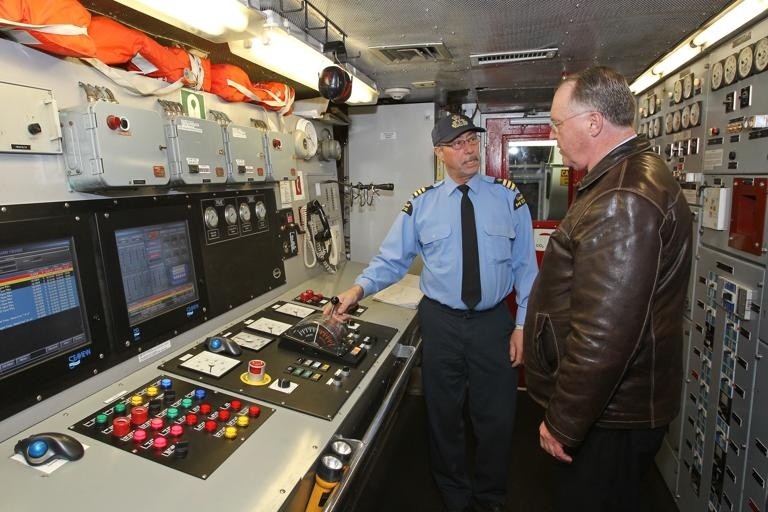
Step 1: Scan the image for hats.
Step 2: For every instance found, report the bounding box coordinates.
[431,113,487,147]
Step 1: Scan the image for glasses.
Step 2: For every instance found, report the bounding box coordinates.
[436,135,481,150]
[549,111,592,132]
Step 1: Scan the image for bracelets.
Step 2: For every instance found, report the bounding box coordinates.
[515,324,525,330]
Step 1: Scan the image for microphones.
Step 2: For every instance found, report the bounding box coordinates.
[361,182,394,191]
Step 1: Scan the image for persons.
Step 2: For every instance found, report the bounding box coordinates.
[321,114,542,510]
[517,63,694,511]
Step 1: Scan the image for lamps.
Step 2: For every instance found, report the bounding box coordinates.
[629,1,768,96]
[227,8,379,105]
[113,0,268,45]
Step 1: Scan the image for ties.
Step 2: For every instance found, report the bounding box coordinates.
[458,184,482,310]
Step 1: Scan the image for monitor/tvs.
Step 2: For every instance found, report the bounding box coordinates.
[112,218,200,327]
[0,236,93,382]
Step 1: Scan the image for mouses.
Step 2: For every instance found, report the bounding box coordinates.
[15,432,84,467]
[204,337,242,357]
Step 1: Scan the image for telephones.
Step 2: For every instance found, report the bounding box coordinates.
[307,200,342,266]
